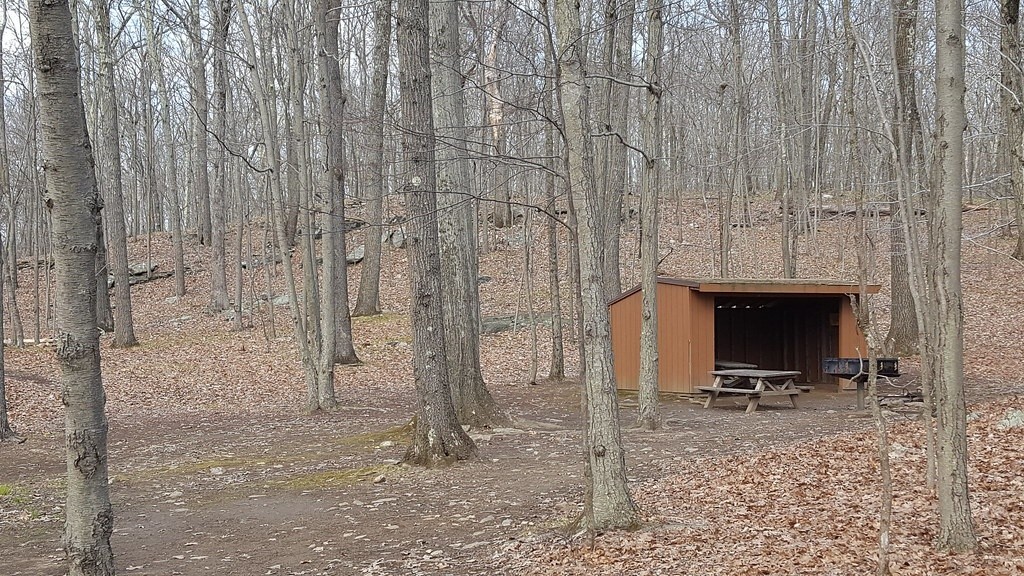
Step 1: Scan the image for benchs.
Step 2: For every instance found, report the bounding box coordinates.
[696,385,763,394]
[750,383,814,393]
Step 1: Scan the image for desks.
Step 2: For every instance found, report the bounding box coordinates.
[716,362,758,389]
[703,369,802,413]
